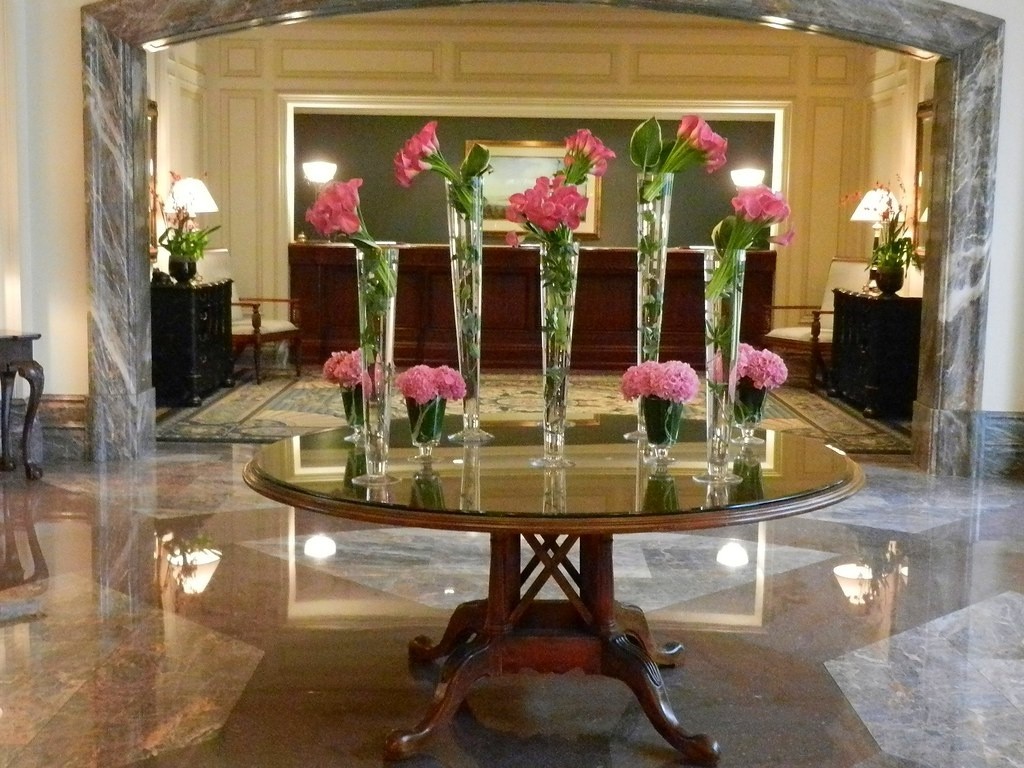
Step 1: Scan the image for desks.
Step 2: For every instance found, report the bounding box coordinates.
[289,241,776,370]
[0,332,45,480]
[243,414,866,765]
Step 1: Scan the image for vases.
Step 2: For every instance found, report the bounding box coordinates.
[170,253,198,285]
[339,177,770,489]
[875,267,904,298]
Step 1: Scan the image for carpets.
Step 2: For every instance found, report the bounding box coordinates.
[155,364,913,455]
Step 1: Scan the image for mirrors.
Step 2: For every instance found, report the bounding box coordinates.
[913,98,935,267]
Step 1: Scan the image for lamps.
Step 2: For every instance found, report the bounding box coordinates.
[833,564,890,606]
[166,548,222,596]
[163,178,219,232]
[302,162,338,200]
[850,189,905,293]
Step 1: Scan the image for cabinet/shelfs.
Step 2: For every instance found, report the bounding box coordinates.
[831,287,923,417]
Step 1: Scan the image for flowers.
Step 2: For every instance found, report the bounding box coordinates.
[150,170,221,260]
[306,114,794,444]
[843,171,922,278]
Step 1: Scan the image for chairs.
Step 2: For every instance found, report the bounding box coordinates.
[196,249,301,386]
[762,256,872,389]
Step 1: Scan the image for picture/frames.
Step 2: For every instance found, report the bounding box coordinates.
[466,140,603,242]
